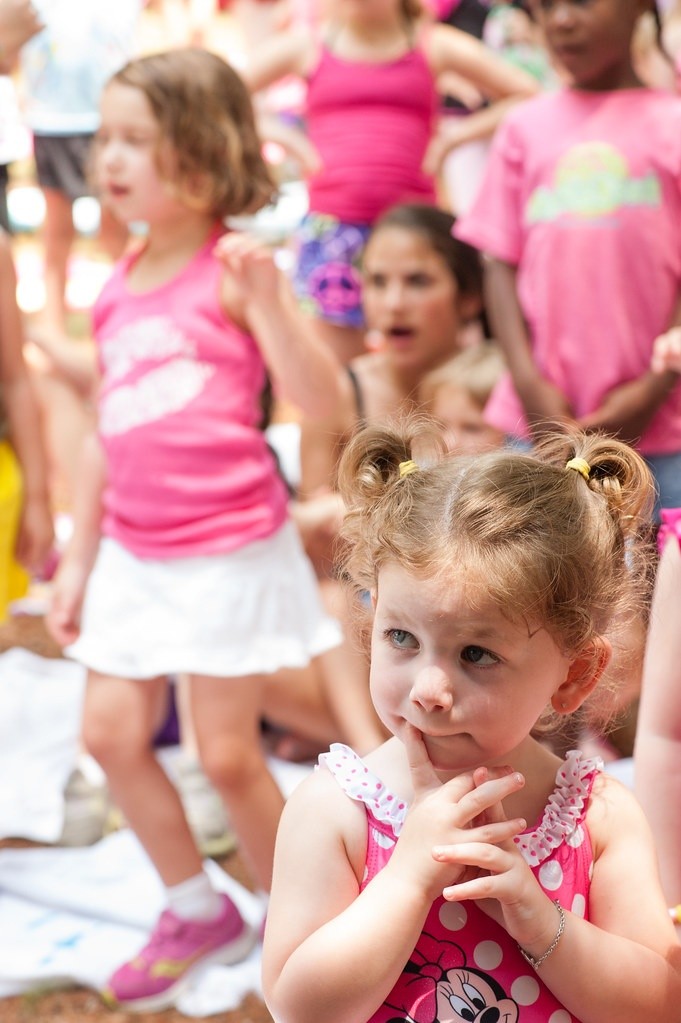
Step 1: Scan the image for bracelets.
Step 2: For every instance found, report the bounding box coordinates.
[519,899,564,970]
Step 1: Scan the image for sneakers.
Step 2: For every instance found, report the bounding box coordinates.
[100,892,256,1015]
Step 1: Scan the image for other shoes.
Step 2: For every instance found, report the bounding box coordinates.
[68,765,128,831]
[176,760,236,856]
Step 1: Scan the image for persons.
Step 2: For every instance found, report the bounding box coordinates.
[0,0,681,764]
[260,416,681,1023]
[38,43,357,999]
